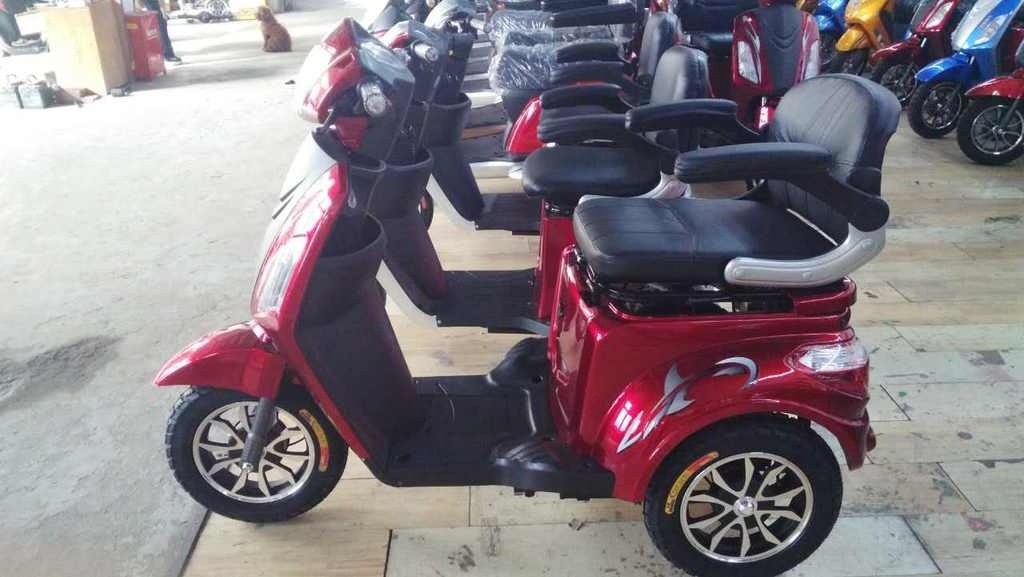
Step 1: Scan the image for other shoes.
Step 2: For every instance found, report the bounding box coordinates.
[168,54,181,62]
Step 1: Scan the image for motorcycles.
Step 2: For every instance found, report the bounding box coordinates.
[144,0,1024,577]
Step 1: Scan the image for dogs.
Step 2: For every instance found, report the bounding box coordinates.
[255,6,291,53]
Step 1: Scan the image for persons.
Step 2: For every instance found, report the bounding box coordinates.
[137,0,182,64]
